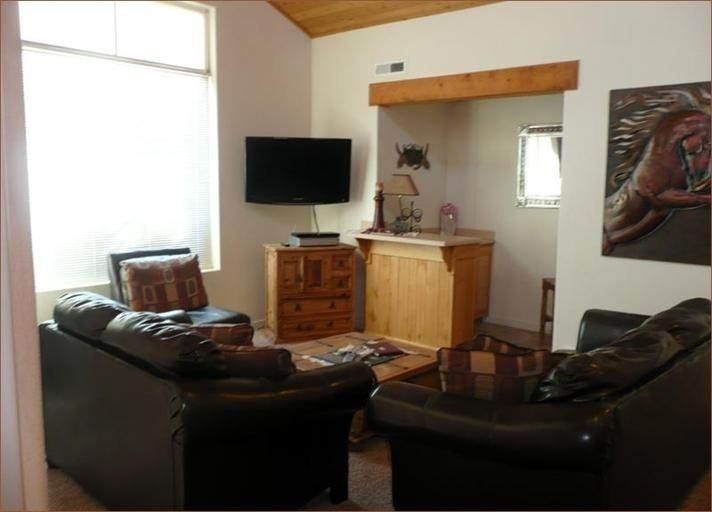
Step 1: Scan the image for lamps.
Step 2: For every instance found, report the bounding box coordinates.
[380,173,420,235]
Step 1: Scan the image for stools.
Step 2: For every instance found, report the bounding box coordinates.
[539,278,555,341]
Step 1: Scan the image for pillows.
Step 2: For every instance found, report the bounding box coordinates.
[118,252,209,312]
[218,344,296,377]
[437,347,551,403]
[179,323,254,346]
[472,334,575,373]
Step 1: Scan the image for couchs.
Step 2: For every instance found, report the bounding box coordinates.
[38,290,377,512]
[106,247,250,324]
[365,297,711,511]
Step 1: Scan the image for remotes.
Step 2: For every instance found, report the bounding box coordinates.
[342,352,356,364]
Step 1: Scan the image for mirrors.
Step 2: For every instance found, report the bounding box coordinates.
[515,124,563,209]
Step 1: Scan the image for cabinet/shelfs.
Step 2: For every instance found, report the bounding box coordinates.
[262,242,357,345]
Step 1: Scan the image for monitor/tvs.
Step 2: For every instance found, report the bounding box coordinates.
[245,135,351,205]
